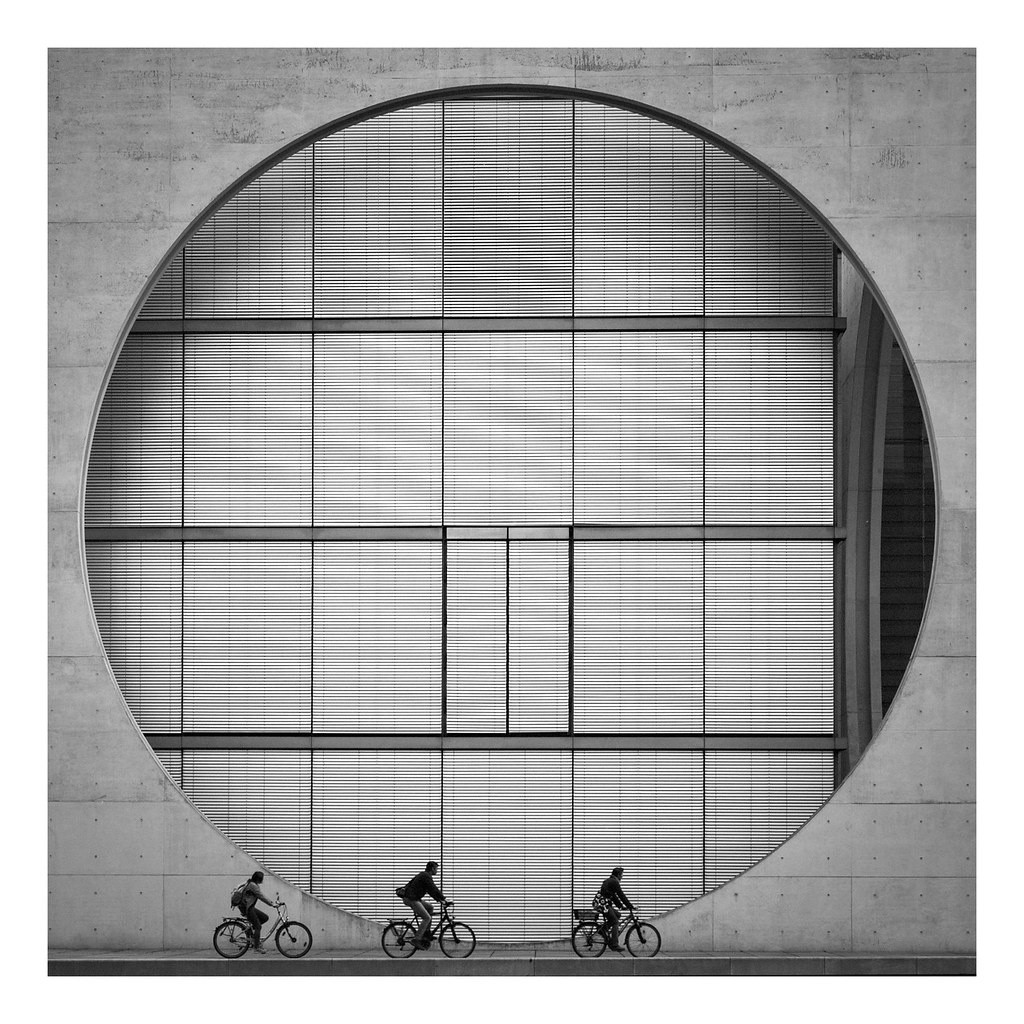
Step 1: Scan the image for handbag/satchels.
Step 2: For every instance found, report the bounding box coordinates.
[593,892,611,915]
[396,886,404,898]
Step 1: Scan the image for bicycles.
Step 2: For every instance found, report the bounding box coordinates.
[381,901,477,959]
[571,906,661,959]
[211,893,314,958]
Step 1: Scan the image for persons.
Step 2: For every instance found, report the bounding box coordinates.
[231,872,279,952]
[592,867,637,951]
[403,860,452,951]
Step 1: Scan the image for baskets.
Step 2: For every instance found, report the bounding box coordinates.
[574,908,599,920]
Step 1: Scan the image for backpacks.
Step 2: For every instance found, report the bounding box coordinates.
[230,879,252,906]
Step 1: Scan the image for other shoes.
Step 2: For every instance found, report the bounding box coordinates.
[410,939,426,950]
[425,937,438,940]
[613,944,625,951]
[254,945,267,954]
